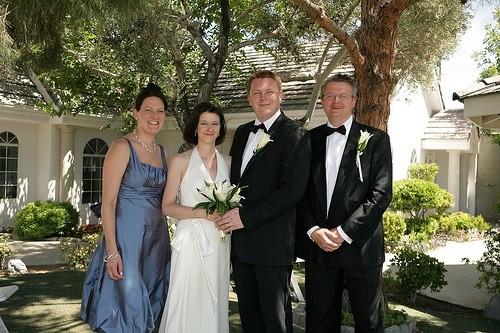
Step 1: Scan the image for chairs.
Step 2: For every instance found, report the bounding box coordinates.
[0,285,19,333]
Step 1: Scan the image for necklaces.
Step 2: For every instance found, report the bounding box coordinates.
[133,129,157,153]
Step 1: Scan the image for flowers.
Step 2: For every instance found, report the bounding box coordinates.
[253,132,274,156]
[355,129,375,153]
[192,178,250,243]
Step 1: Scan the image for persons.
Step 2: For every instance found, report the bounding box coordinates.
[214,71,307,333]
[296,73,393,333]
[80,83,171,333]
[158,102,231,333]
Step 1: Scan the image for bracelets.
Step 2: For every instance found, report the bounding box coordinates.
[104,253,119,262]
[107,252,118,259]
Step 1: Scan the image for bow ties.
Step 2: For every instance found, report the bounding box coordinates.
[325,125,346,136]
[250,123,268,134]
